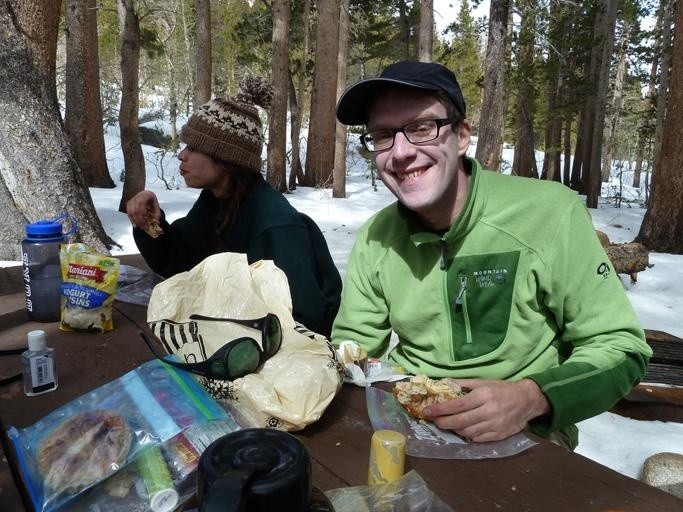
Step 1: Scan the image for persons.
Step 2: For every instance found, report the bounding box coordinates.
[331,63,654,453]
[127,98,342,338]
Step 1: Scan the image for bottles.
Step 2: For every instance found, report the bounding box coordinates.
[20,330,59,396]
[22,215,79,321]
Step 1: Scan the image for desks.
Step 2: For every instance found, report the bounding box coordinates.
[0,252,683,511]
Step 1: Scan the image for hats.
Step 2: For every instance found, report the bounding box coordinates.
[180,76,276,173]
[337,62,466,125]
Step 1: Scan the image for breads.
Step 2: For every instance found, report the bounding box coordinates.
[34,410,132,494]
[395,375,464,419]
[139,209,164,239]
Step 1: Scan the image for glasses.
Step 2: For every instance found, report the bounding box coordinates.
[142,312,283,379]
[358,115,463,152]
